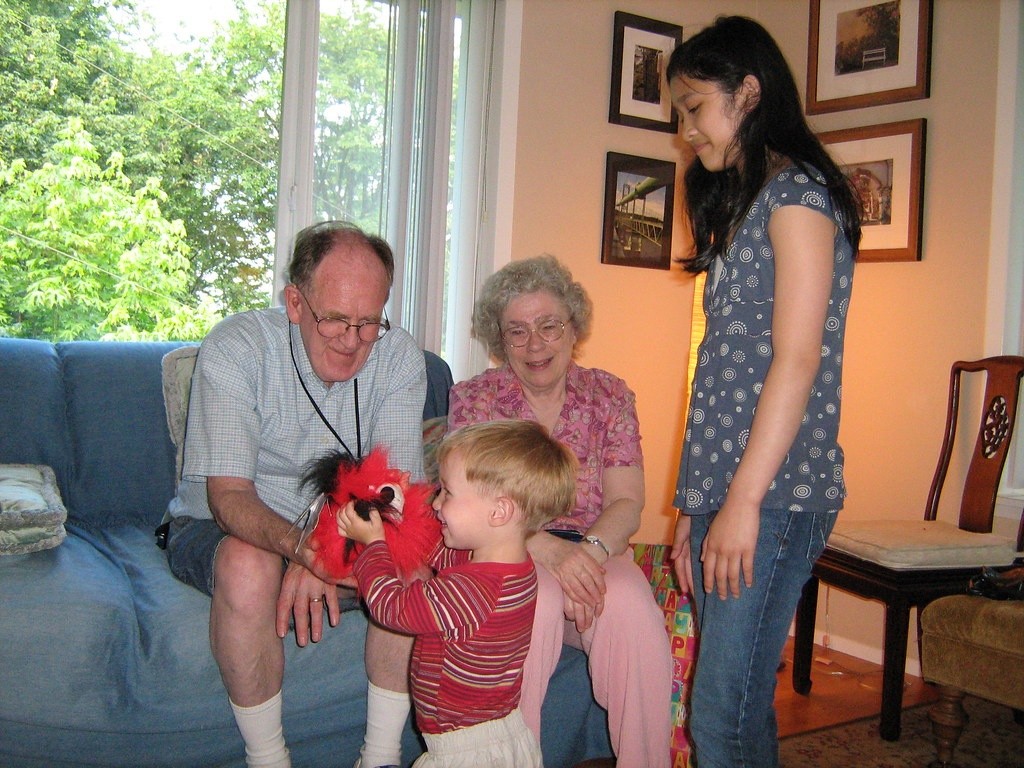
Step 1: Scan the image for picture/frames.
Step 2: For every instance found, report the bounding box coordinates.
[815,118,928,263]
[606,10,684,135]
[804,0,931,117]
[601,151,676,270]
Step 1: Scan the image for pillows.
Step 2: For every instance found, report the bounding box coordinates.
[0,463,68,555]
[163,346,201,501]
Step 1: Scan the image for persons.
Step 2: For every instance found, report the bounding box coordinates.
[165,221,432,767]
[447,251,672,768]
[665,22,865,767]
[334,417,579,768]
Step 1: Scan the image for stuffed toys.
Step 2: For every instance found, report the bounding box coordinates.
[299,438,446,585]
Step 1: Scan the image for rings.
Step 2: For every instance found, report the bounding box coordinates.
[309,598,323,603]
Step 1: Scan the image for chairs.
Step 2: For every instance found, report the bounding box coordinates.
[790,356,1024,738]
[920,590,1024,768]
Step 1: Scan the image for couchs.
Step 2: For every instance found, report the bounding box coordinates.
[0,334,614,768]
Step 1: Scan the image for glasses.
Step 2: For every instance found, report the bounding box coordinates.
[290,283,390,342]
[499,316,574,347]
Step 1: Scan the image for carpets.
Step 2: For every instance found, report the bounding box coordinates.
[772,694,1024,768]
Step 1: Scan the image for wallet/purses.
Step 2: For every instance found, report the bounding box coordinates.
[545,529,583,539]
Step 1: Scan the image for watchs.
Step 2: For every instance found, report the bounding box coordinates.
[580,536,610,559]
